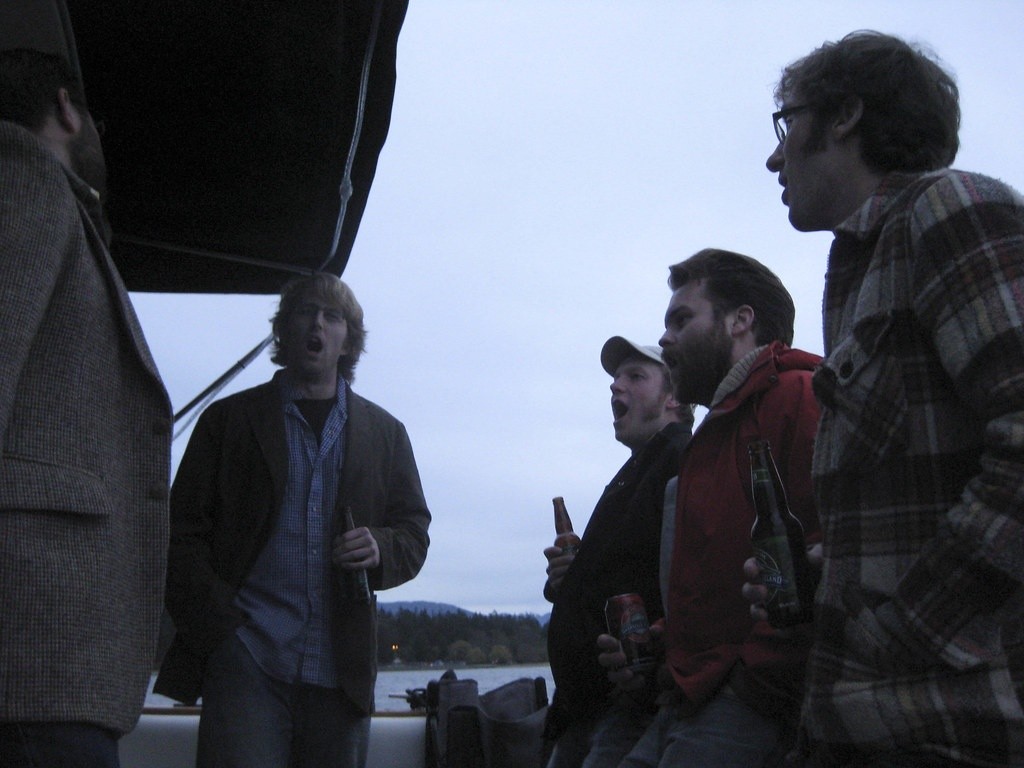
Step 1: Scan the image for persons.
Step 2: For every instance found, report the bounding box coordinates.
[151,273,433,768]
[543,333,695,768]
[741,30,1024,768]
[0,47,175,765]
[598,246,823,768]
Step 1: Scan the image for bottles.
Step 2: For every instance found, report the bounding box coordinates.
[345,506,374,604]
[552,495,582,561]
[749,436,815,630]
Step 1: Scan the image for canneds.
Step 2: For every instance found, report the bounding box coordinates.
[604,593,657,671]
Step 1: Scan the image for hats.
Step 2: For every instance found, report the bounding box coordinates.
[601,336,667,377]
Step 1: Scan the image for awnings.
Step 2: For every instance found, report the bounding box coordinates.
[0,1,404,425]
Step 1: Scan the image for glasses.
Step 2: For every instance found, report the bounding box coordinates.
[773,102,832,144]
